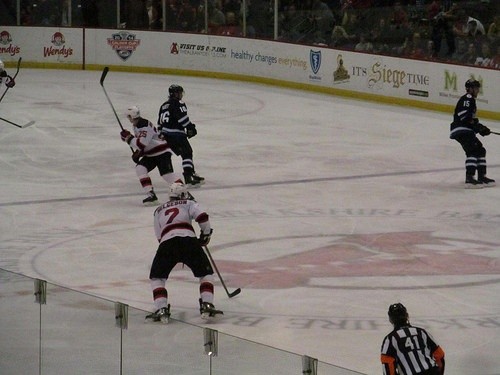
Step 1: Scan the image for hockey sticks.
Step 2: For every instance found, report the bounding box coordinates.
[0,117,36,129]
[0,57,21,100]
[203,244,241,298]
[100,67,135,155]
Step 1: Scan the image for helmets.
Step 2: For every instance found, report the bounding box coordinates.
[464,78,480,88]
[168,84,184,94]
[126,106,140,119]
[388,302,407,323]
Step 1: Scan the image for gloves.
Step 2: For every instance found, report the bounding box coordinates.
[6,77,15,89]
[132,153,140,163]
[186,130,198,139]
[120,130,132,142]
[480,126,491,137]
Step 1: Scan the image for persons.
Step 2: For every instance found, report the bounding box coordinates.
[156,85,205,188]
[0,59,9,85]
[121,105,187,204]
[381,303,445,375]
[146,181,225,321]
[1,2,499,69]
[450,79,495,190]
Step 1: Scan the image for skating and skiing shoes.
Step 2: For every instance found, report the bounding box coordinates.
[184,192,196,202]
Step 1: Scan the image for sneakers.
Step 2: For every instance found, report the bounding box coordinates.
[477,176,496,186]
[142,192,160,207]
[145,303,171,324]
[183,173,206,189]
[169,181,187,198]
[197,298,224,321]
[464,175,483,189]
